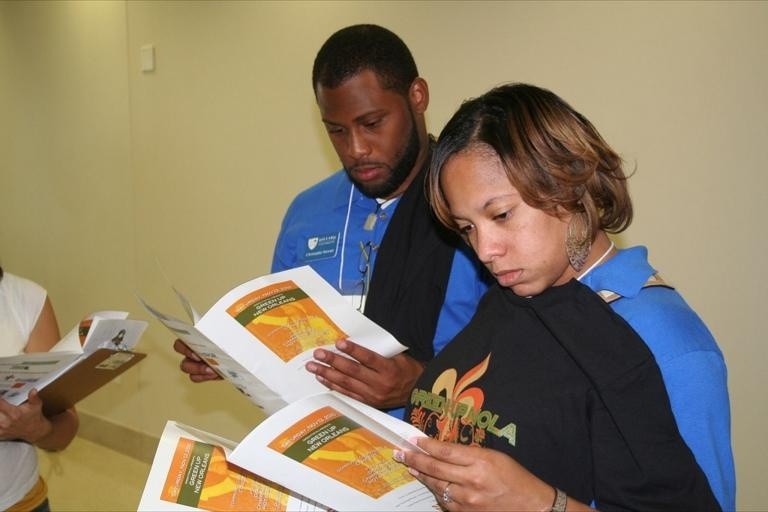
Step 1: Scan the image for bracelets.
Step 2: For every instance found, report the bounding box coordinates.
[551,486,568,511]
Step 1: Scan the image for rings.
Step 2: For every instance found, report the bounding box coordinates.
[442,481,452,504]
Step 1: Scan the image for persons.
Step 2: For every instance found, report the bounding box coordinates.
[174,22,494,421]
[391,81,737,512]
[1,269,80,511]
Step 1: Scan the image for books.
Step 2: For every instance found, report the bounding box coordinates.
[140,263,407,415]
[0,310,149,417]
[138,390,442,512]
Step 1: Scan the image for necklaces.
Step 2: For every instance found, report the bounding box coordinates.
[576,238,615,283]
